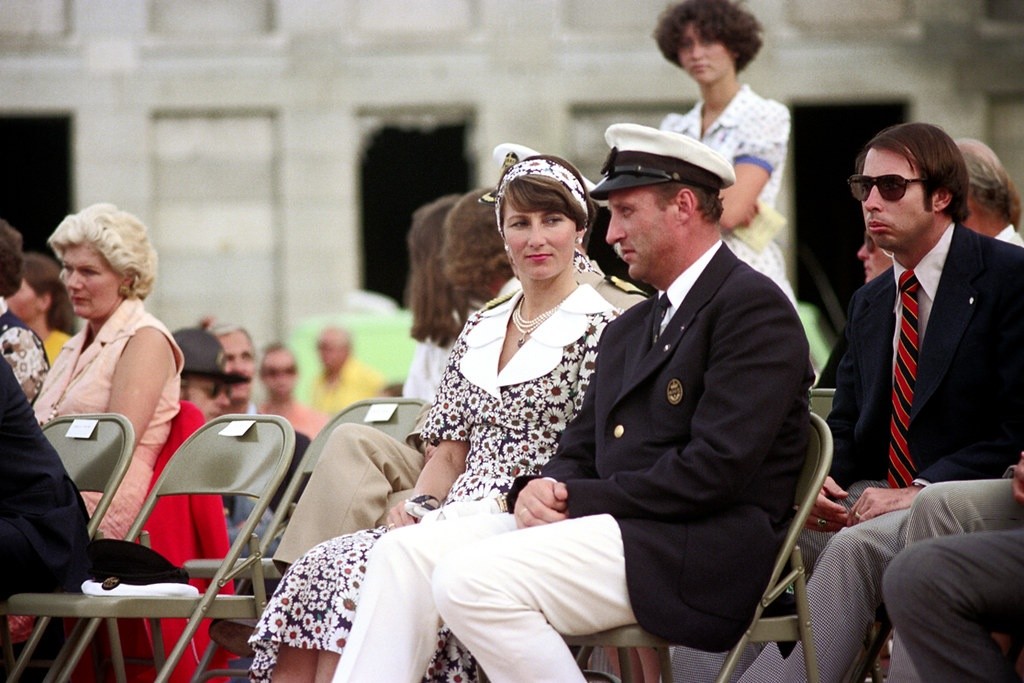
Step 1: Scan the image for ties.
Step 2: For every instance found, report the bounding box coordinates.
[886,268,920,491]
[652,292,671,345]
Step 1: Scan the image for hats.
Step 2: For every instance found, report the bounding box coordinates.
[589,123,737,201]
[80,539,200,600]
[171,329,250,384]
[478,143,611,208]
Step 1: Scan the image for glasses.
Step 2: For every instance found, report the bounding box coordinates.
[863,232,877,253]
[180,379,234,398]
[847,175,924,201]
[269,366,295,377]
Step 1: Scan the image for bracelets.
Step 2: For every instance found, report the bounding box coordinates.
[494,494,508,514]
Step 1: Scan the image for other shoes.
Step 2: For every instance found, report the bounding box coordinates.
[208,617,262,658]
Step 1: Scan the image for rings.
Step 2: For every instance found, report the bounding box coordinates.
[517,508,528,517]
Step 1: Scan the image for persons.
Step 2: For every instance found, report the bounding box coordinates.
[653,2,796,304]
[0,201,403,683]
[331,121,818,683]
[493,142,647,317]
[406,187,461,403]
[242,153,622,683]
[675,123,1024,683]
[444,186,504,311]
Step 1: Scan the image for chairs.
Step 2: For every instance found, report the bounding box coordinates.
[0,388,891,683]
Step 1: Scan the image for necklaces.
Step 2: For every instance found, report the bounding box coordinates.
[508,296,566,347]
